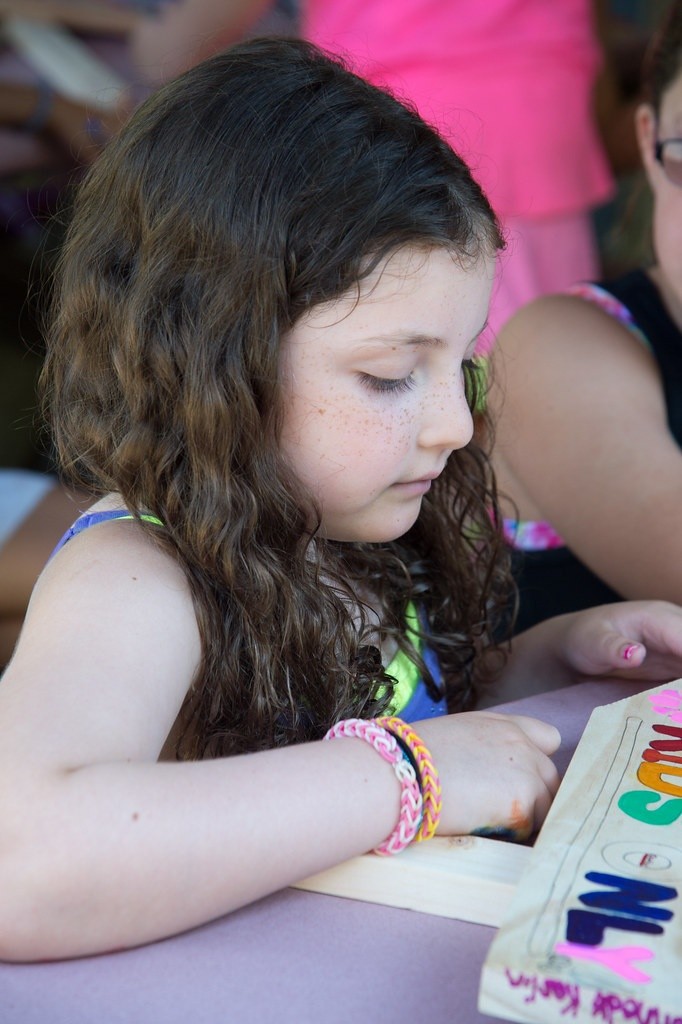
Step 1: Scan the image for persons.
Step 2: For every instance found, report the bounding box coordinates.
[0,1,289,667]
[463,2,677,639]
[0,32,680,967]
[290,0,620,400]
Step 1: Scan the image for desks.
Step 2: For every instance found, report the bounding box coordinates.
[2,672,660,1024]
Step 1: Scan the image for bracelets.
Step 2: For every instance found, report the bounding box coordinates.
[322,710,443,860]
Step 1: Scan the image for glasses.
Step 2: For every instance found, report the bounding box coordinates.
[651,114,682,187]
